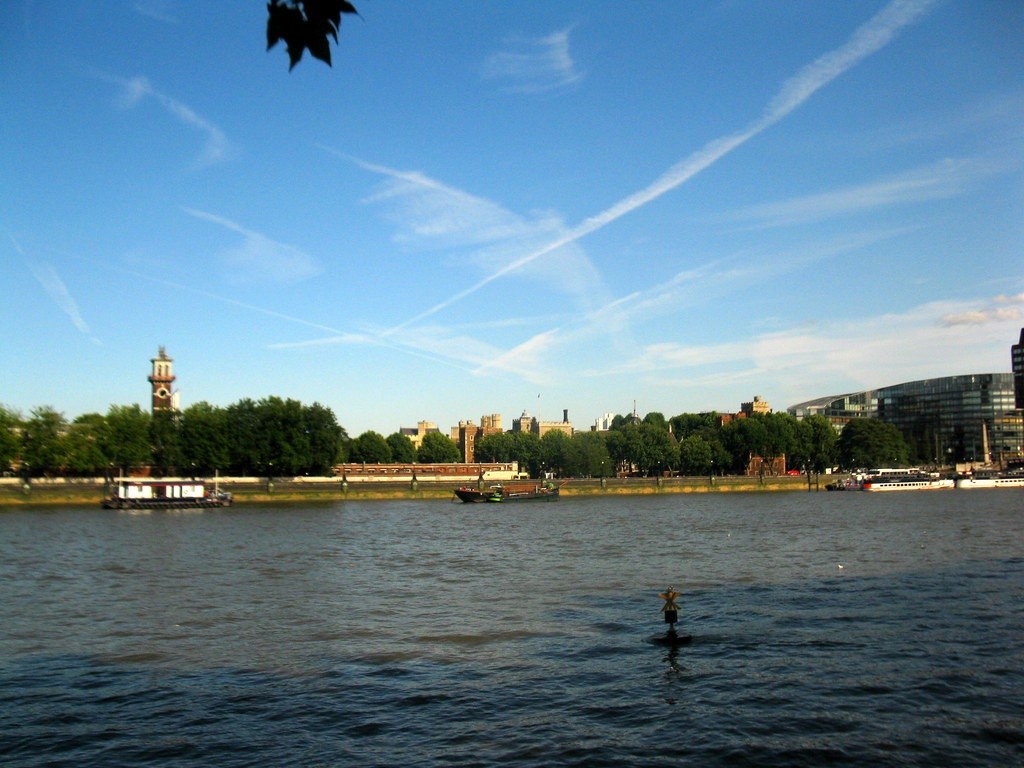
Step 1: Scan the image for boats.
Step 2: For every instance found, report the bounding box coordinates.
[454,471,560,503]
[100,480,235,509]
[863,477,955,490]
[956,476,1024,488]
